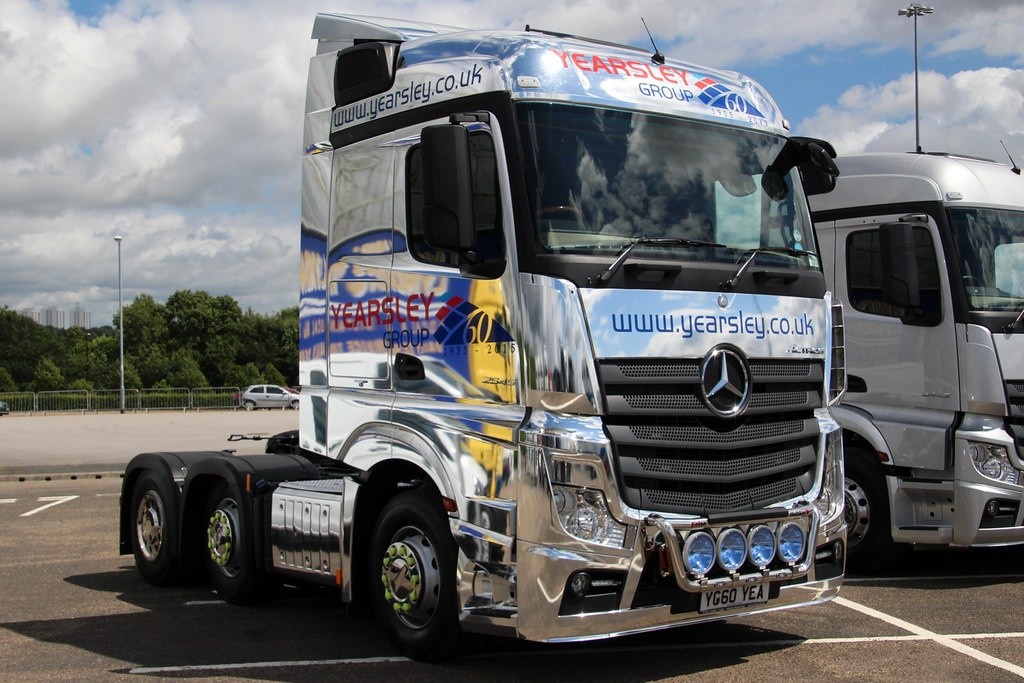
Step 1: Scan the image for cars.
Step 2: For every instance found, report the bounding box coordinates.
[241,384,300,411]
[0,402,9,417]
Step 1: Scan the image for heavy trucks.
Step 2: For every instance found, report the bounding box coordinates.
[807,151,1024,579]
[117,11,848,672]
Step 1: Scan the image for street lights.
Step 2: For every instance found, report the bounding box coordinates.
[112,235,125,413]
[897,2,934,154]
